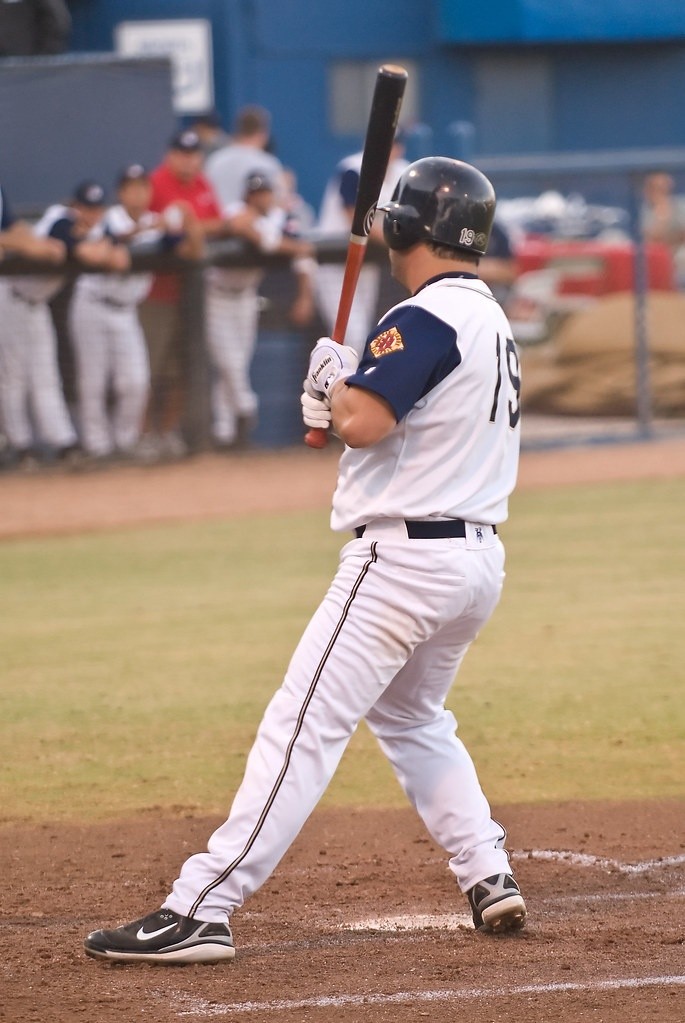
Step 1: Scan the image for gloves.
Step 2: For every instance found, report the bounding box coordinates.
[300,379,332,428]
[308,337,358,399]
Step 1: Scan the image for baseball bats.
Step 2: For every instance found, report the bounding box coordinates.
[299,60,410,453]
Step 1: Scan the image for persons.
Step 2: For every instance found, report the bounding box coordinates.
[0,107,409,470]
[82,155,528,963]
[640,169,685,246]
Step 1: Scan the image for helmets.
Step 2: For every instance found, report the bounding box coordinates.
[377,158,496,256]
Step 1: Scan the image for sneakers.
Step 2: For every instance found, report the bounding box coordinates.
[83,909,235,965]
[467,873,528,933]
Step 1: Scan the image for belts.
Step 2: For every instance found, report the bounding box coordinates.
[356,519,498,539]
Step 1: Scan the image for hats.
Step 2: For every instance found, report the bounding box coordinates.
[76,181,108,206]
[115,163,146,181]
[172,130,204,153]
[245,171,270,196]
